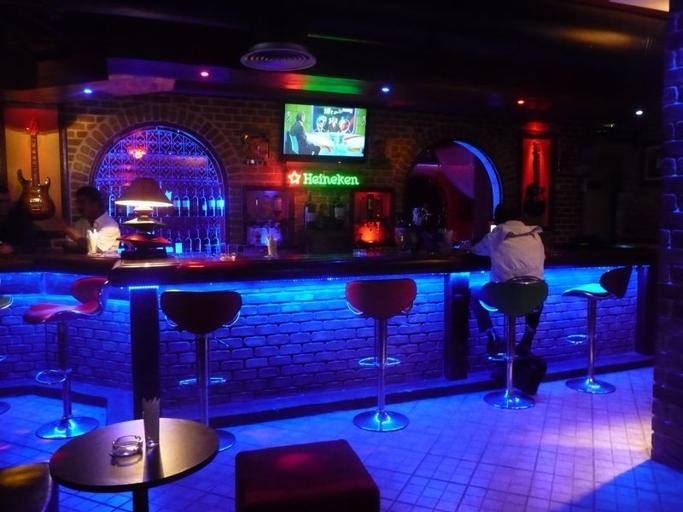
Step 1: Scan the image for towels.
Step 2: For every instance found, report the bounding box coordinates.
[266,234,278,256]
[142,396,160,442]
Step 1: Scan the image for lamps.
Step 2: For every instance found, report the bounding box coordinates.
[114,177,175,266]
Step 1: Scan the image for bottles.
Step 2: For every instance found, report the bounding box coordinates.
[190,188,198,216]
[108,185,116,218]
[192,229,201,254]
[182,230,192,253]
[116,185,126,216]
[173,189,180,217]
[366,193,375,220]
[273,196,283,217]
[215,187,223,216]
[174,231,182,254]
[126,205,134,218]
[332,196,345,221]
[211,228,220,254]
[198,187,207,216]
[207,186,215,216]
[304,190,318,225]
[165,230,173,253]
[201,229,211,253]
[180,188,190,217]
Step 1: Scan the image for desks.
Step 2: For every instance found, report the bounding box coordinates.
[50,416,219,512]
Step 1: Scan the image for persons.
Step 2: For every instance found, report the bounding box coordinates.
[62,186,121,256]
[470,199,548,357]
[287,111,320,157]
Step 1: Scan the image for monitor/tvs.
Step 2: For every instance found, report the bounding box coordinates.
[279,101,370,164]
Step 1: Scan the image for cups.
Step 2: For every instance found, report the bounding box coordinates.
[139,409,163,449]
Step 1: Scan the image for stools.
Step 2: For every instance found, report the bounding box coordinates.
[478,276,549,411]
[161,290,243,452]
[236,438,381,507]
[0,463,57,508]
[0,295,14,413]
[345,279,417,433]
[561,265,635,395]
[23,277,110,440]
